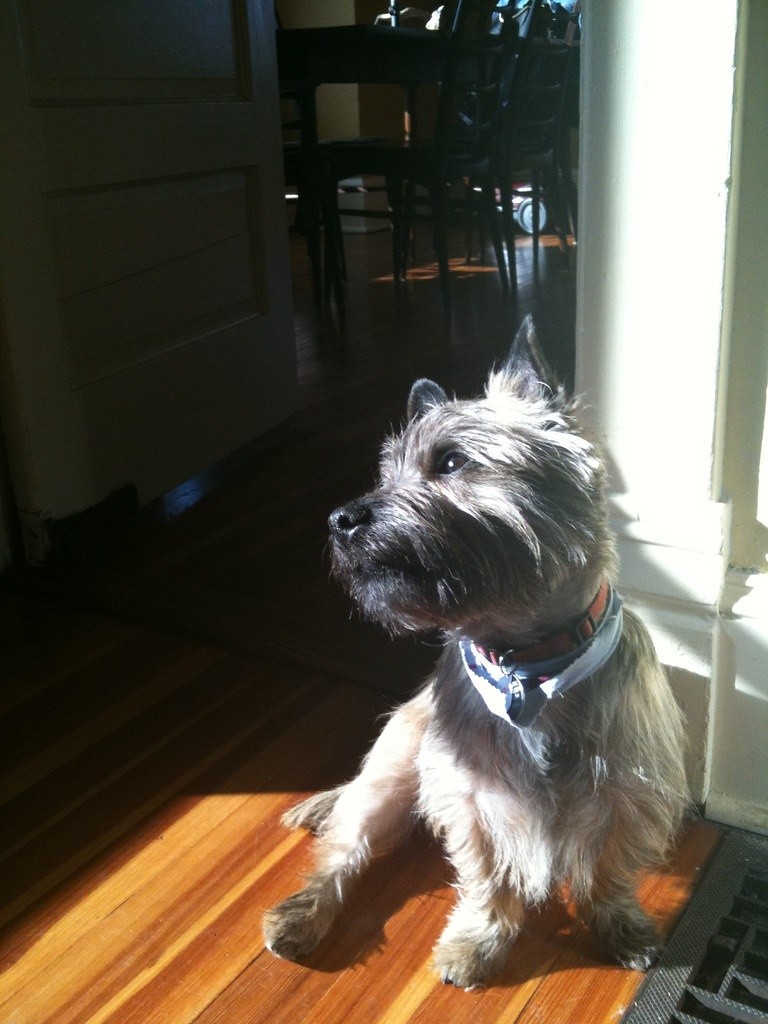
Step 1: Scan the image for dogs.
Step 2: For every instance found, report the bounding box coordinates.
[256,327,685,984]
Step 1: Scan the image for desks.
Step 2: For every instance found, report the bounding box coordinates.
[275,24,581,314]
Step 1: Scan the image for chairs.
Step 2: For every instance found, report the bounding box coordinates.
[283,0,580,336]
